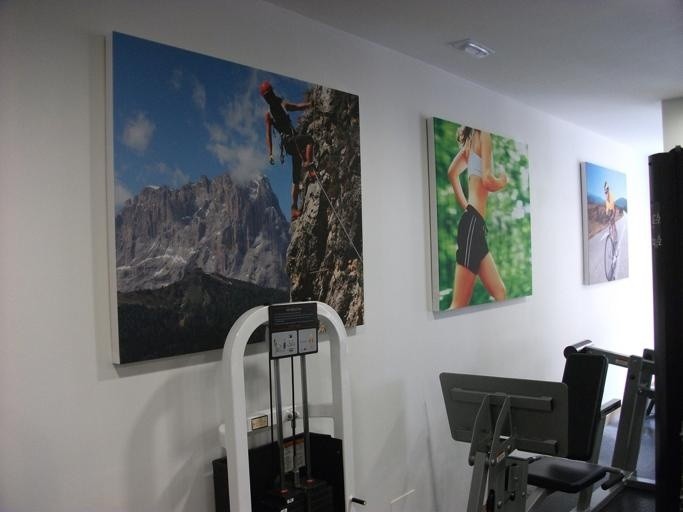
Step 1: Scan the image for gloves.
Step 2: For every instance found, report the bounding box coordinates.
[269,153,274,165]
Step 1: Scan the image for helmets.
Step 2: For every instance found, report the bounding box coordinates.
[260,81,273,96]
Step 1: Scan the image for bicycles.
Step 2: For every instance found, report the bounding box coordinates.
[601,215,619,281]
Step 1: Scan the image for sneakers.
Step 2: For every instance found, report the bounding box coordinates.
[291,210,303,221]
[308,171,319,178]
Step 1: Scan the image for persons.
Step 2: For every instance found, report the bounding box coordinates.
[258,80,315,219]
[604,182,617,255]
[445,124,508,310]
